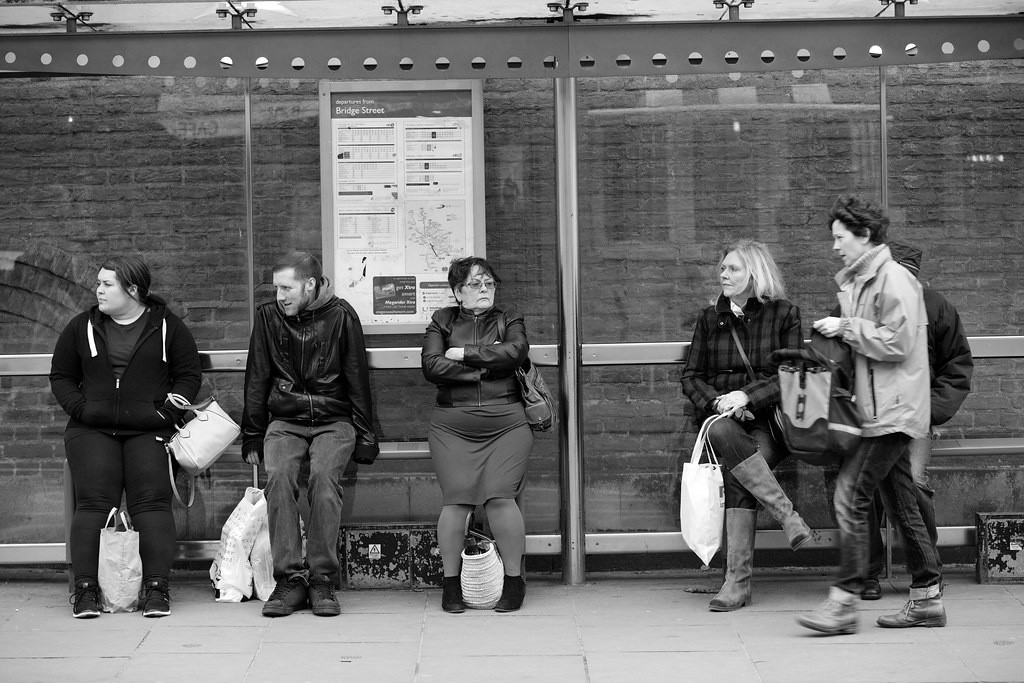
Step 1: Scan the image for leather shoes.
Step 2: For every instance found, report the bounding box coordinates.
[861,579,883,601]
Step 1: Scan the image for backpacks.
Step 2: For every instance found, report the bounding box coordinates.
[773,331,861,465]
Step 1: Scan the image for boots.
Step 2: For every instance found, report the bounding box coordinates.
[709,507,757,611]
[496,576,527,612]
[730,452,810,552]
[442,575,466,613]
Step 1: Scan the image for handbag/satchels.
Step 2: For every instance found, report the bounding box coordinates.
[163,393,243,510]
[209,486,268,604]
[97,507,144,613]
[251,513,307,600]
[461,510,503,611]
[680,410,731,566]
[499,311,555,431]
[727,316,786,441]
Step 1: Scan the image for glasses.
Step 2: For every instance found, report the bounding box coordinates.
[463,280,498,289]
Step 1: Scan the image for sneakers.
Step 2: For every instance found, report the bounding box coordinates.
[308,584,341,615]
[262,577,306,616]
[137,574,173,616]
[69,579,100,618]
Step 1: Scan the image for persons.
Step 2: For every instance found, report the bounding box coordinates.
[48,256,200,617]
[420,256,535,615]
[241,249,381,616]
[681,238,813,612]
[797,194,948,634]
[827,240,975,600]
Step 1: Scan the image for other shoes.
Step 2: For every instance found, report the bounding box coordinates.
[876,599,948,628]
[794,587,858,635]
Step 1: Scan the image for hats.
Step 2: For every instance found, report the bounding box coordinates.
[886,240,922,278]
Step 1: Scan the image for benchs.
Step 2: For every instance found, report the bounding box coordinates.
[723,437,1024,585]
[65,442,524,593]
